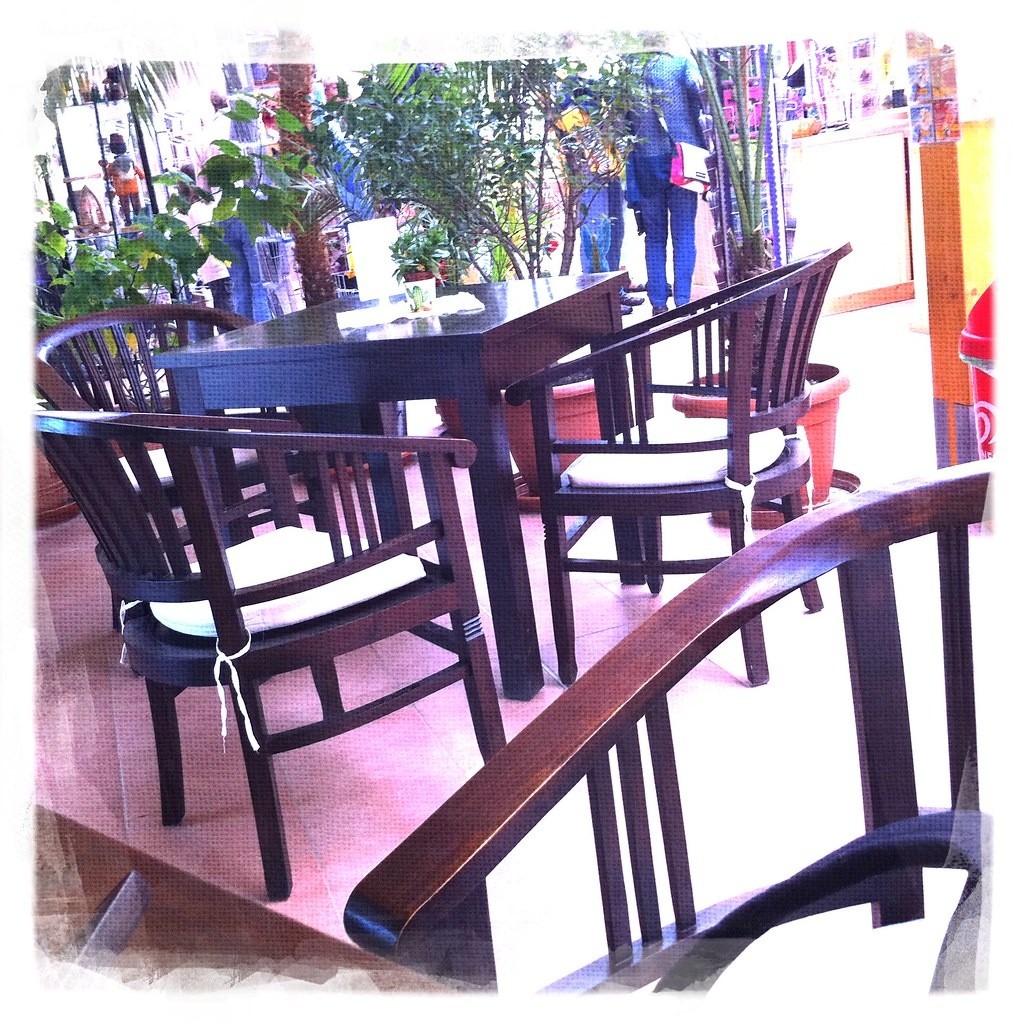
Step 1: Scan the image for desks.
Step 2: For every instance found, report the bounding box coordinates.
[152,272,648,700]
[35,806,474,991]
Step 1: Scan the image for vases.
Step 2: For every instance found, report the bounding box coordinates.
[107,397,177,458]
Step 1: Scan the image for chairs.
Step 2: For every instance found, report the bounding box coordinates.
[345,459,993,995]
[505,242,852,687]
[35,411,508,903]
[35,303,334,631]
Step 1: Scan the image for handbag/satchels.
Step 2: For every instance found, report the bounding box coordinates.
[669,141,710,196]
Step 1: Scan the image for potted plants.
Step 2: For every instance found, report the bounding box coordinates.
[33,43,849,526]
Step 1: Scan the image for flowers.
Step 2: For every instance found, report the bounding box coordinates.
[34,78,357,414]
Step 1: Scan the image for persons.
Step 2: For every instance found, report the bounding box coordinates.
[102,133,146,224]
[554,47,708,317]
[179,90,269,332]
[323,75,350,107]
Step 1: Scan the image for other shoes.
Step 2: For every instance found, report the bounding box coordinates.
[619,303,634,315]
[619,293,644,306]
[653,306,668,318]
